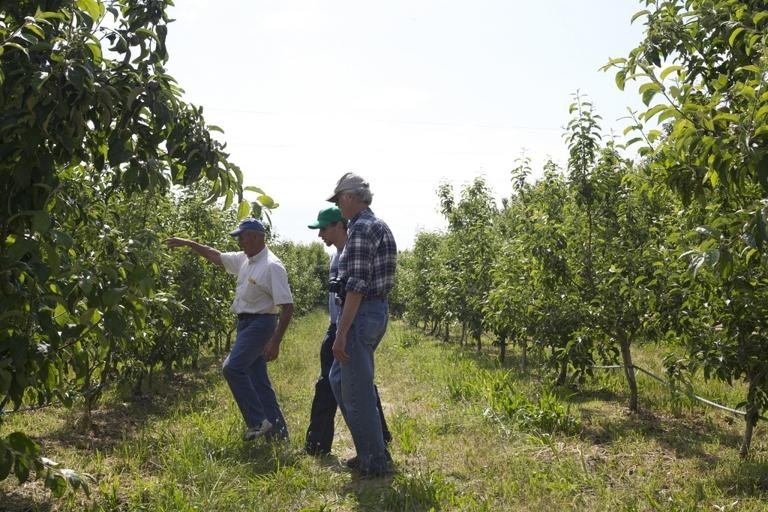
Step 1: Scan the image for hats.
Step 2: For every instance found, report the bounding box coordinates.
[229,218,264,235]
[325,171,368,202]
[307,207,343,229]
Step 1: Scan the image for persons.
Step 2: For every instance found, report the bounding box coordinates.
[297,206,393,457]
[160,218,296,449]
[323,171,397,474]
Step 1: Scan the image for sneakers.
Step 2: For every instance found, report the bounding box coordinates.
[243,418,273,441]
[347,447,393,470]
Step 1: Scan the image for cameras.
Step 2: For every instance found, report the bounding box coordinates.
[328,277,347,306]
